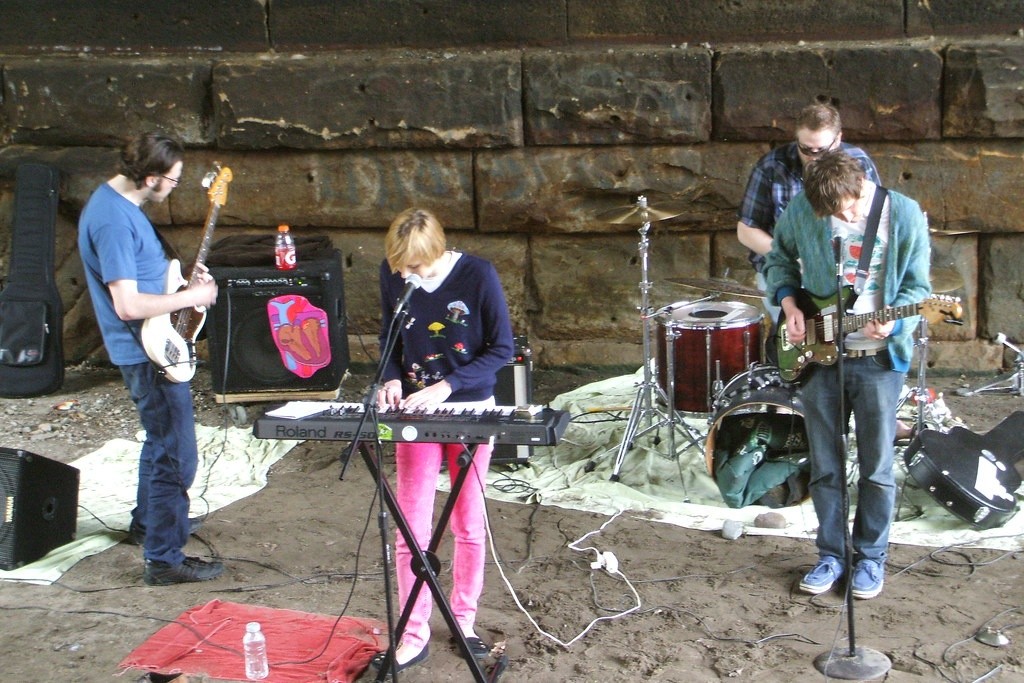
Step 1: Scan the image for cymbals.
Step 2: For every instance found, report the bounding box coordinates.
[595,204,689,225]
[663,277,770,299]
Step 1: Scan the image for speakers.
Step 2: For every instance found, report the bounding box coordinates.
[209,248,350,394]
[0,447,81,571]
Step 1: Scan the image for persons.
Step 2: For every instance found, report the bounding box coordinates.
[372,207,514,674]
[737,104,882,323]
[762,150,932,599]
[78,131,224,585]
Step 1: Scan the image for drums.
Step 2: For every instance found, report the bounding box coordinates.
[702,364,816,509]
[655,302,764,421]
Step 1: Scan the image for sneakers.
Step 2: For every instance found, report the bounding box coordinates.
[129,517,202,543]
[145,557,223,586]
[800,555,845,595]
[851,558,885,599]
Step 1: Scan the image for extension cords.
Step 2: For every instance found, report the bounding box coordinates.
[603,552,618,573]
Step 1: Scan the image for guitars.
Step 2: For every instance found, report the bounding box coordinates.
[776,285,965,384]
[139,160,234,384]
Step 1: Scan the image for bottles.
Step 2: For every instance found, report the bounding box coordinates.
[274,224,296,271]
[243,622,269,680]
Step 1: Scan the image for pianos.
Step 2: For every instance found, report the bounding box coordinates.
[254,397,571,683]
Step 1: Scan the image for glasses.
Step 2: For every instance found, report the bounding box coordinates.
[161,175,180,187]
[797,137,833,158]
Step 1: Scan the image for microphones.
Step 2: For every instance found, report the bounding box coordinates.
[835,237,842,283]
[392,274,422,318]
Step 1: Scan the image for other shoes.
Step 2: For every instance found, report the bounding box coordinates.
[371,643,430,676]
[465,628,488,658]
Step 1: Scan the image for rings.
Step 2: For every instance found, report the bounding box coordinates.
[381,389,387,391]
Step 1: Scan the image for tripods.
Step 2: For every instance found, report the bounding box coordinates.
[583,196,729,503]
[846,317,948,489]
